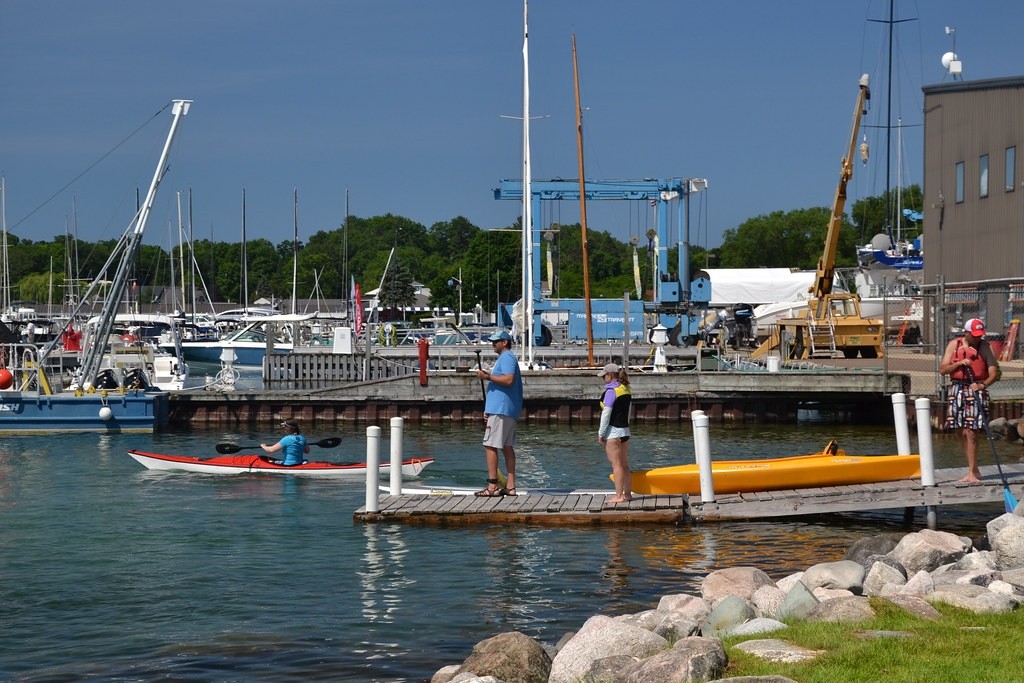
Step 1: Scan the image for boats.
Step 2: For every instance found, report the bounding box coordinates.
[126,448,436,483]
[608,439,921,495]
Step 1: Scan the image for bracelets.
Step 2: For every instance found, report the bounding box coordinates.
[486,374,490,381]
[980,382,986,389]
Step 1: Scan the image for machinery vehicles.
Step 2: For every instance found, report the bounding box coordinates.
[749,78,886,360]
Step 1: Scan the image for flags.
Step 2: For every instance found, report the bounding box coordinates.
[351,275,362,334]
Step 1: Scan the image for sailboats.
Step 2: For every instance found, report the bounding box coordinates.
[1,1,695,394]
[744,3,923,343]
[0,99,195,440]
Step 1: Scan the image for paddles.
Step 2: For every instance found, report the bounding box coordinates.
[965,365,1019,514]
[474,348,507,488]
[215,437,342,455]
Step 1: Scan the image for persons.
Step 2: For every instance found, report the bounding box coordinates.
[939,318,998,483]
[260,420,310,466]
[474,330,523,497]
[596,363,632,503]
[27,322,34,344]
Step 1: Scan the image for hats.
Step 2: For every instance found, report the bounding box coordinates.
[597,363,620,377]
[964,318,987,337]
[488,330,512,341]
[280,421,297,429]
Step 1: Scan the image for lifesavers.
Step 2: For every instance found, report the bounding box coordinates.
[120,334,133,342]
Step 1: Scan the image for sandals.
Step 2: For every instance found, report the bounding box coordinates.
[474,488,500,497]
[500,486,516,496]
